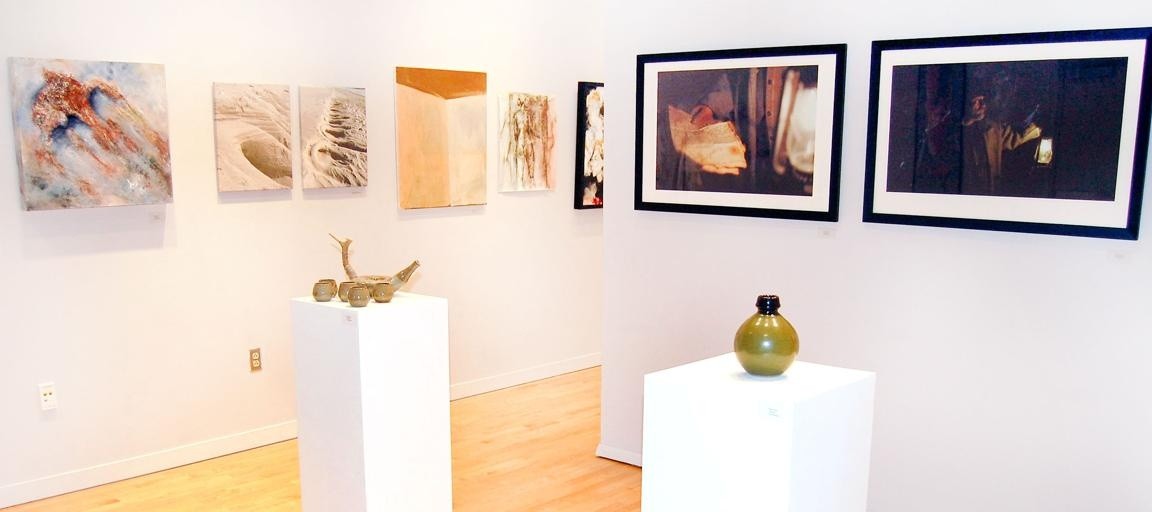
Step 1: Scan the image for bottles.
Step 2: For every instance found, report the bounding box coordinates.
[732,292,800,377]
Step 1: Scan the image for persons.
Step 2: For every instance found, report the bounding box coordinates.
[772,66,818,197]
[924,88,1041,195]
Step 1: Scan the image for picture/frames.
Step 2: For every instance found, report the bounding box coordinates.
[861,27,1152,241]
[633,43,847,222]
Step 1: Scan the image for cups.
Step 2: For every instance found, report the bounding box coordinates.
[349,287,368,306]
[337,280,355,301]
[373,282,394,302]
[313,282,332,302]
[320,279,337,295]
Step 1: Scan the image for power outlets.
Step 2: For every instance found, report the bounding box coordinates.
[38,382,56,411]
[249,347,262,372]
